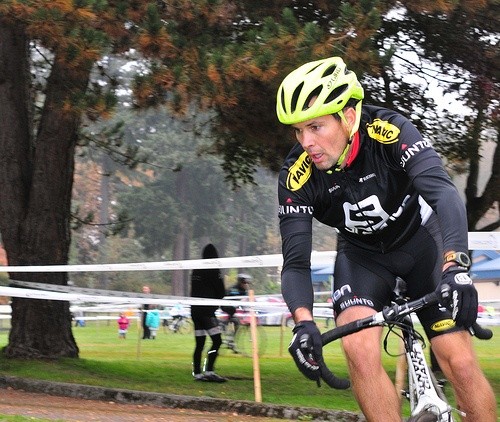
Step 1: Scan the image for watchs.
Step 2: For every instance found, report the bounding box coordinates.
[443,251,471,269]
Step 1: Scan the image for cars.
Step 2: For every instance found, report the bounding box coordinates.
[217,297,297,328]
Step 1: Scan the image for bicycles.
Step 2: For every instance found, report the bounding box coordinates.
[214,311,267,358]
[161,313,195,334]
[296,286,493,417]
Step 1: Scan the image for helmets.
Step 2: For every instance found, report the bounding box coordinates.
[276,56,364,125]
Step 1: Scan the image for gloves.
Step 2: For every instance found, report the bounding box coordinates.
[439,264,478,331]
[288,321,323,381]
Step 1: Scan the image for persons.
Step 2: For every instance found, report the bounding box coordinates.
[136,285,191,340]
[275,57,497,422]
[75,320,85,327]
[117,313,130,339]
[190,243,236,382]
[219,273,253,354]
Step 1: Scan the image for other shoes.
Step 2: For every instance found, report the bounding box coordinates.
[192,372,209,382]
[205,371,229,383]
